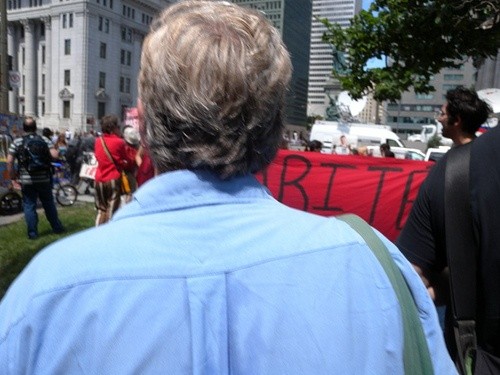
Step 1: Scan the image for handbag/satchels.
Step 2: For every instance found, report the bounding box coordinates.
[121,171,137,194]
[454,347,500,375]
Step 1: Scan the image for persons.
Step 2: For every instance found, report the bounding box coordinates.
[6,117,67,240]
[39,125,155,196]
[94,115,130,227]
[394,119,500,375]
[0,0,459,375]
[281,129,395,159]
[438,85,493,145]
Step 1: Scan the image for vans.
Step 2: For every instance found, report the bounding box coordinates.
[309,119,406,163]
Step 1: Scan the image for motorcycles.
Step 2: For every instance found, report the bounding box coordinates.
[0,156,77,216]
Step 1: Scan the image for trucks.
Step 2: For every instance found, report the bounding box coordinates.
[409,118,456,144]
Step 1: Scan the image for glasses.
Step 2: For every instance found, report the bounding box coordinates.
[438,109,449,116]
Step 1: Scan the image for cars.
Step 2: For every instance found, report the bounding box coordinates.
[365,144,427,162]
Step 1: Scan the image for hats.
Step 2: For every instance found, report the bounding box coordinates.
[124,127,141,145]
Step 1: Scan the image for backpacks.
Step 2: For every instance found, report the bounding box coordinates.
[16,134,55,176]
